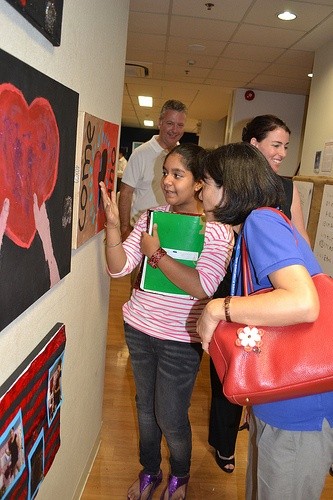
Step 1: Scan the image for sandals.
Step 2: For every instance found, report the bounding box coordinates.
[128,469,164,500]
[161,473,191,500]
[216,447,236,474]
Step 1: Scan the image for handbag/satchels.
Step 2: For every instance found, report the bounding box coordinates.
[208,206,333,406]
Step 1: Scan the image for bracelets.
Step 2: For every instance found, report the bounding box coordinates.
[225,296,232,322]
[103,239,123,247]
[104,222,121,229]
[147,248,167,269]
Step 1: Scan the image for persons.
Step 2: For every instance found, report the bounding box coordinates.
[118,100,187,292]
[100,143,234,500]
[208,115,310,474]
[196,143,332,500]
[117,150,127,194]
